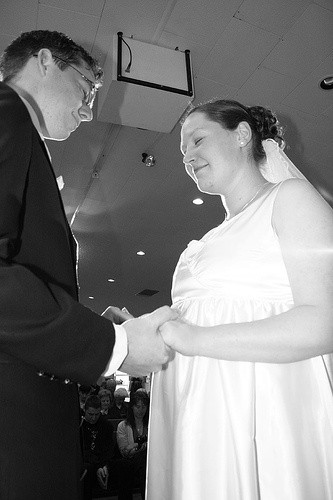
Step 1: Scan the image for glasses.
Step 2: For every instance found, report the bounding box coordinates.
[33,54,97,110]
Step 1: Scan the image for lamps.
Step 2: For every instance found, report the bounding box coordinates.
[141,152,155,167]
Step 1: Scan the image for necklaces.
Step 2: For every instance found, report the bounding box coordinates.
[225,181,269,221]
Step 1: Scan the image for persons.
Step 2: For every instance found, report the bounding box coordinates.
[0,30,180,500]
[77,377,149,431]
[116,391,150,500]
[79,396,132,500]
[145,99,332,500]
